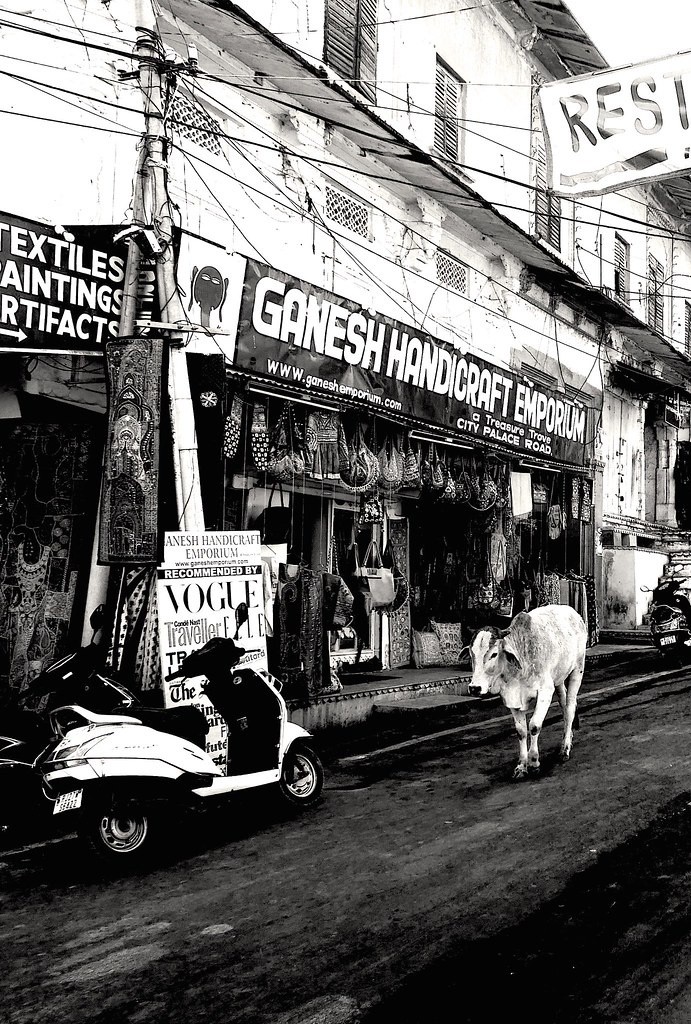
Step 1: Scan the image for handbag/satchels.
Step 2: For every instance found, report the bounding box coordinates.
[547,472,564,540]
[265,395,511,615]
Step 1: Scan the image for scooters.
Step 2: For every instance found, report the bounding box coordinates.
[41,601,323,862]
[0,603,150,851]
[639,563,691,656]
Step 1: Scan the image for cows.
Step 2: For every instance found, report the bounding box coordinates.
[460,604,588,780]
